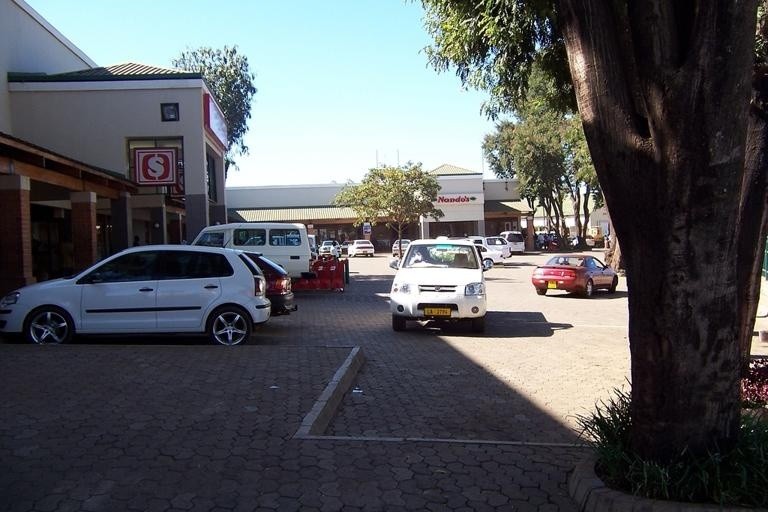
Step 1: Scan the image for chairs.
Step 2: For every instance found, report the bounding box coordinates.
[449,254,469,268]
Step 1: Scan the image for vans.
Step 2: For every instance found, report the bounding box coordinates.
[189,222,318,284]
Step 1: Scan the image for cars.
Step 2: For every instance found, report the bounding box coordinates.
[244,234,316,252]
[1,245,271,346]
[531,254,618,299]
[245,250,298,317]
[392,239,411,257]
[469,231,526,266]
[318,240,342,258]
[348,240,375,258]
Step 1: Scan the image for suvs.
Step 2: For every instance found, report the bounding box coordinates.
[388,240,494,332]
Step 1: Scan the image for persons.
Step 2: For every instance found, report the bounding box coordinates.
[410,249,432,265]
[533,233,553,251]
[133,234,141,246]
[330,241,340,258]
